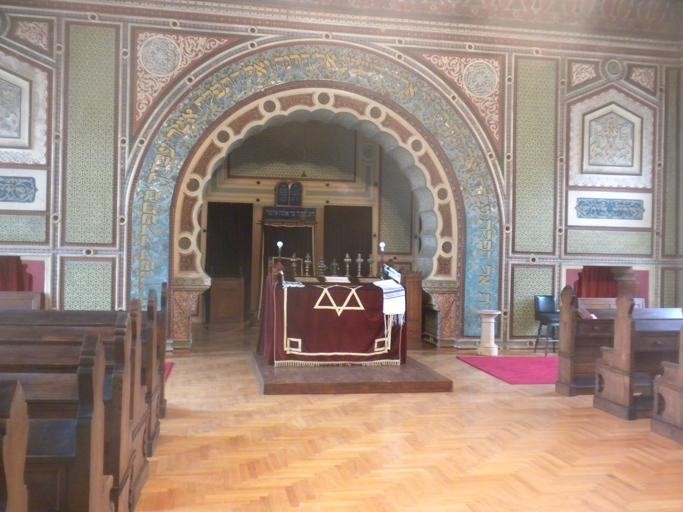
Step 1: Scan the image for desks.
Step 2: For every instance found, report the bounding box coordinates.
[257,263,406,367]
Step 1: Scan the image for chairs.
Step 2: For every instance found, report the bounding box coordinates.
[532,293,561,356]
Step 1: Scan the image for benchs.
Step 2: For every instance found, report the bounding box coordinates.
[0,276,173,512]
[553,282,683,452]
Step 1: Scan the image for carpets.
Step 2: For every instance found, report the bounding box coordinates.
[454,352,561,386]
